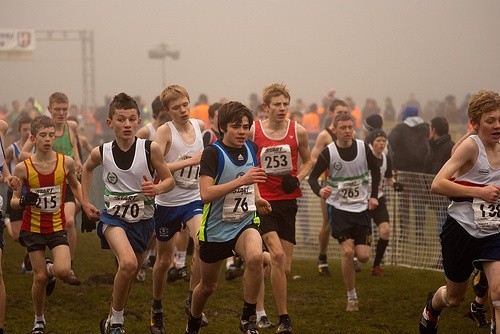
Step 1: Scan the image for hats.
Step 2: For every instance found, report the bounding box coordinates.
[362,114,383,132]
[366,128,388,144]
[400,106,418,120]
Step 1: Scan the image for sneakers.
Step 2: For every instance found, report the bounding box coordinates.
[30,316,46,334]
[149,310,165,334]
[21,252,385,285]
[418,292,440,334]
[275,319,292,334]
[101,307,125,334]
[346,296,359,314]
[468,300,488,328]
[254,316,275,328]
[182,324,201,334]
[238,316,259,334]
[44,257,56,296]
[185,302,208,325]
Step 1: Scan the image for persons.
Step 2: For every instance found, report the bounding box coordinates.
[183,101,272,334]
[449,90,498,334]
[309,113,381,312]
[10,115,100,334]
[0,132,20,334]
[243,82,314,334]
[419,88,500,334]
[1,85,483,282]
[82,91,176,334]
[150,85,210,334]
